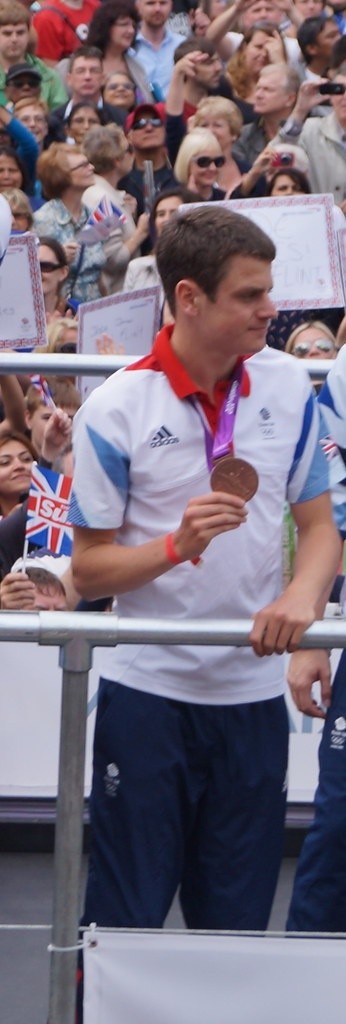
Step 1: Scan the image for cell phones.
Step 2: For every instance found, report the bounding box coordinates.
[319,83,346,94]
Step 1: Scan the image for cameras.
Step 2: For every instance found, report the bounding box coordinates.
[271,151,294,167]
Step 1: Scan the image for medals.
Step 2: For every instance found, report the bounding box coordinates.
[210,458,258,503]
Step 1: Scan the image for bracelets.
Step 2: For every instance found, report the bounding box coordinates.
[164,532,202,567]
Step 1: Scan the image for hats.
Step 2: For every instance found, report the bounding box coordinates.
[124,104,167,130]
[5,63,43,81]
[321,35,346,77]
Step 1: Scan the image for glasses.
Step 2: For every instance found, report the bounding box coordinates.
[108,83,133,90]
[292,340,333,358]
[70,159,91,172]
[131,117,162,129]
[124,142,134,155]
[192,155,226,168]
[13,80,40,87]
[40,262,62,272]
[11,209,27,220]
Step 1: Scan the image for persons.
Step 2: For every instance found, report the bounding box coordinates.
[287,338,344,932]
[1,0,346,610]
[69,205,344,1022]
